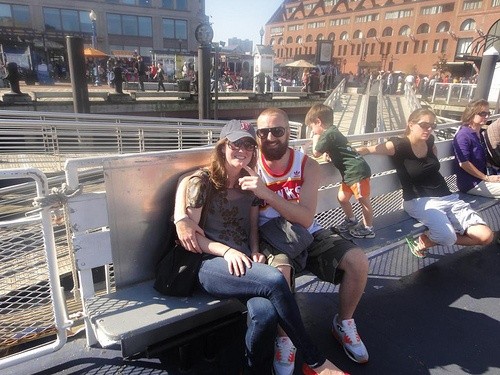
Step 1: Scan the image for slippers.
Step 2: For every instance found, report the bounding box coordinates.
[405,237,427,259]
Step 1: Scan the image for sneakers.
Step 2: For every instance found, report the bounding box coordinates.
[330,218,359,233]
[273,337,297,375]
[332,313,369,364]
[350,223,376,239]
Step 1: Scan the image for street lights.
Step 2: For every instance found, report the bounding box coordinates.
[89,8,101,88]
[40,25,50,71]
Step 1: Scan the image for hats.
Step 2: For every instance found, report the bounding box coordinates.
[219,120,257,145]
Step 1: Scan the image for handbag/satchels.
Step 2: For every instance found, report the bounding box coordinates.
[152,239,205,297]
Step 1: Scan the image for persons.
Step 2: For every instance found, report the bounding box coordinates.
[175,121,349,375]
[305,103,375,237]
[183,62,198,94]
[292,69,326,92]
[173,108,369,375]
[86,56,166,92]
[452,99,500,243]
[325,108,493,258]
[347,71,466,95]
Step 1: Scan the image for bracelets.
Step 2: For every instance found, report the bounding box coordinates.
[223,247,231,257]
[174,216,188,224]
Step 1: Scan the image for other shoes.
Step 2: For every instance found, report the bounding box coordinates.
[301,364,349,375]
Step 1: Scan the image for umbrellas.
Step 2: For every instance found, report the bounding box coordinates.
[84,47,104,57]
[286,59,316,78]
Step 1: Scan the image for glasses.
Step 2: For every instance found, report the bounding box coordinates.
[255,127,286,140]
[473,111,490,117]
[417,122,437,130]
[225,139,255,151]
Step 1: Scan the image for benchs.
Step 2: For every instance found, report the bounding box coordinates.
[84,113,500,375]
